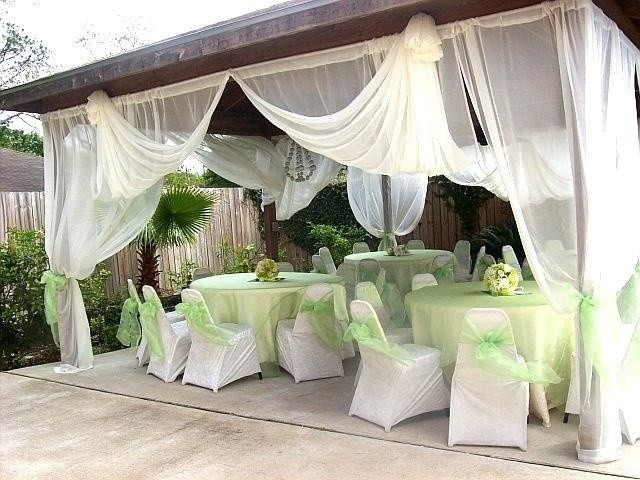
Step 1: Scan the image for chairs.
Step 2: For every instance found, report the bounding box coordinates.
[358,258,407,328]
[352,241,371,255]
[407,238,426,250]
[329,283,356,362]
[318,246,337,275]
[563,334,579,425]
[347,299,451,433]
[275,282,346,384]
[335,262,357,313]
[429,254,452,283]
[452,239,472,275]
[311,254,329,274]
[180,288,262,394]
[446,307,531,452]
[501,244,524,283]
[275,261,295,273]
[476,253,497,282]
[191,266,213,281]
[141,284,192,384]
[472,245,487,281]
[355,280,413,345]
[127,278,186,367]
[411,272,438,292]
[377,237,397,252]
